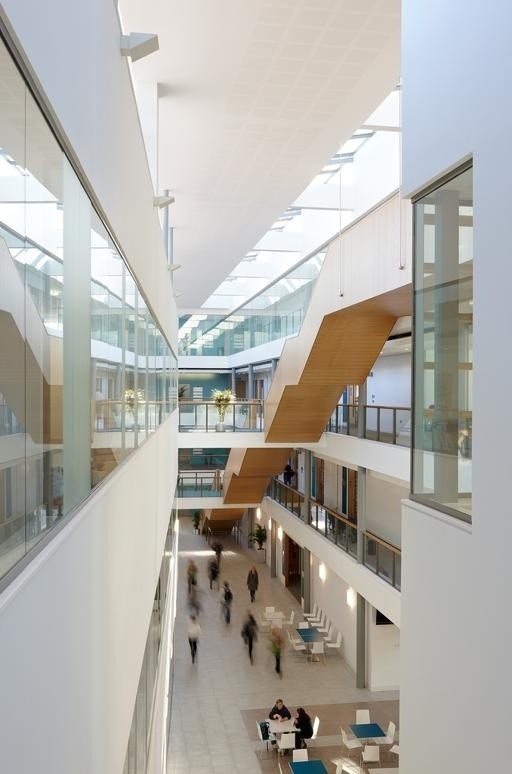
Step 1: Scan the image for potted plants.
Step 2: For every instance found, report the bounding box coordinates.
[247,523,268,563]
[124,388,145,431]
[211,387,235,431]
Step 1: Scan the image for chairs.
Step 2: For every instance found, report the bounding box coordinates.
[258,604,344,667]
[255,708,400,774]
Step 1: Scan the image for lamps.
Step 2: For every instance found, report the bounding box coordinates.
[154,195,174,209]
[120,31,158,62]
[168,264,182,271]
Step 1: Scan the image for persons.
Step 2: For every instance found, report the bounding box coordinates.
[246,566,259,602]
[187,560,197,594]
[295,707,313,749]
[220,580,233,623]
[268,626,284,675]
[208,539,224,590]
[283,464,293,486]
[187,616,201,663]
[269,699,291,754]
[241,614,259,666]
